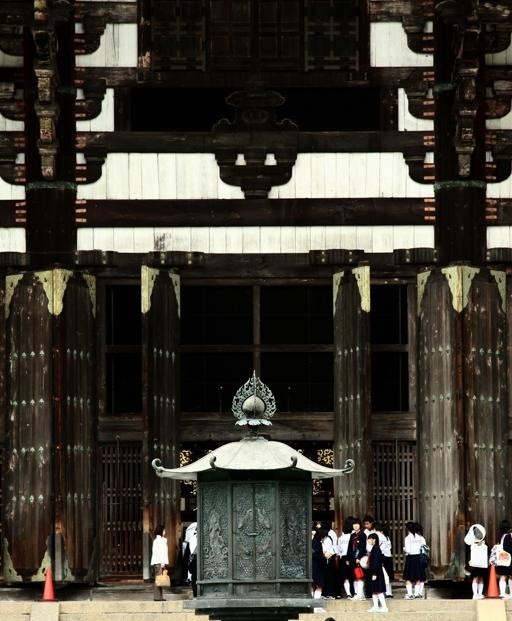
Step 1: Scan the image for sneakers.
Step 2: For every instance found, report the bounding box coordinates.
[403,593,422,599]
[347,593,366,601]
[473,594,484,600]
[368,606,388,612]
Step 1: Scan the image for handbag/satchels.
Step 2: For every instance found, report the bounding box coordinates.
[489,544,511,567]
[155,567,170,587]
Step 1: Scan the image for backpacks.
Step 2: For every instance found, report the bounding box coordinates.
[420,545,430,567]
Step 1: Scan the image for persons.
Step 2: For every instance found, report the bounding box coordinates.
[309,511,394,602]
[150,526,171,601]
[366,533,388,614]
[490,521,511,599]
[401,522,423,601]
[413,523,431,598]
[462,523,489,600]
[181,516,199,601]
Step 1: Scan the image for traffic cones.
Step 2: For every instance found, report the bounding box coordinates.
[39,564,59,601]
[485,562,504,599]
